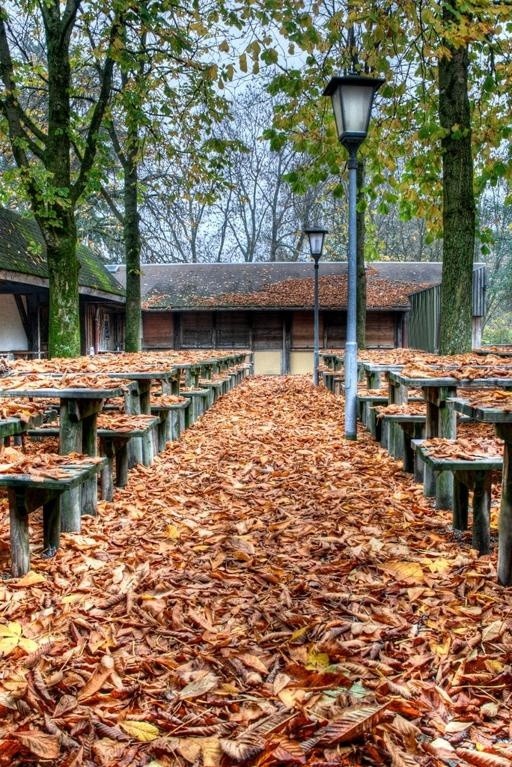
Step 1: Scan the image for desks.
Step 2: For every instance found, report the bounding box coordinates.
[0,350,251,467]
[317,349,511,459]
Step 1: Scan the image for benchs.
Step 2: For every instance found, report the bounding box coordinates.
[317,372,511,586]
[0,378,226,579]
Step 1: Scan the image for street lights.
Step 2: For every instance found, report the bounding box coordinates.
[304,226,329,387]
[322,74,387,440]
